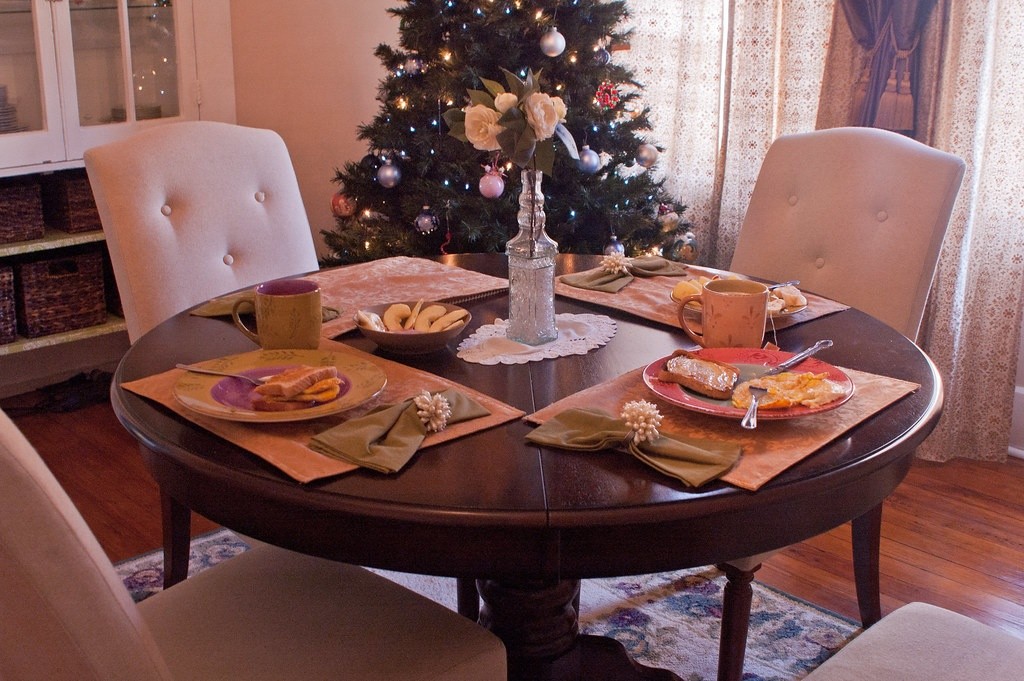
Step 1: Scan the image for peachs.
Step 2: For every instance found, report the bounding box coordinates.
[673,275,740,306]
[358,297,468,334]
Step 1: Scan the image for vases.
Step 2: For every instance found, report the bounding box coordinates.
[505,167,558,346]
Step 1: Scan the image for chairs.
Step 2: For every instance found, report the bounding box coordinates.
[706,126,968,681]
[0,407,509,681]
[801,600,1024,681]
[82,118,320,343]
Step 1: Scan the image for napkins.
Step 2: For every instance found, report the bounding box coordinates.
[190,291,343,318]
[311,385,490,476]
[525,406,744,488]
[559,256,690,294]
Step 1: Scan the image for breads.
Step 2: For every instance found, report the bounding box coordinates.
[659,349,740,400]
[767,283,808,315]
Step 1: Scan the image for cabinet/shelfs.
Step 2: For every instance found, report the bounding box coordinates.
[0,0,237,399]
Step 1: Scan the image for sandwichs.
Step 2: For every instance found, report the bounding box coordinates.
[250,366,342,410]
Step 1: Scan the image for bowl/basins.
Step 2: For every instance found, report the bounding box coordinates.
[351,300,473,352]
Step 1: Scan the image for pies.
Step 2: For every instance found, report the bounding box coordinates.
[732,372,846,409]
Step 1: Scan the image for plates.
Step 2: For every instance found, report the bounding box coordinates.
[642,346,856,419]
[670,277,808,316]
[170,345,390,424]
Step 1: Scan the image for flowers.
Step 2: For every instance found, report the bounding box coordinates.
[441,66,582,192]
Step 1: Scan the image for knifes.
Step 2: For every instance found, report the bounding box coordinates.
[757,338,833,379]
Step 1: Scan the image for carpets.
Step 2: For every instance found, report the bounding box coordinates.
[114,529,862,681]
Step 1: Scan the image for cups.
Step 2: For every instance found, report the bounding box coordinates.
[233,279,321,352]
[677,280,769,351]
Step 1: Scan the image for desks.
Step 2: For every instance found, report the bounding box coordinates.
[109,254,945,681]
